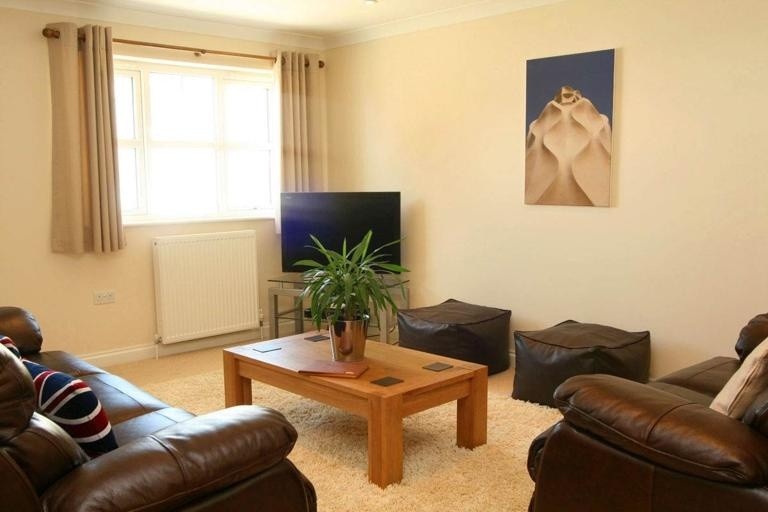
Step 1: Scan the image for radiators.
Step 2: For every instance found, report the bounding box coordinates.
[153,230,263,344]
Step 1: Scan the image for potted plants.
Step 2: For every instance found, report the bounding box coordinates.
[293,230,411,363]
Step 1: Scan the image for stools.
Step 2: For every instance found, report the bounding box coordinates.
[397,300,511,376]
[512,319,651,411]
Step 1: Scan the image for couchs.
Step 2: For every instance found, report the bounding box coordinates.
[527,281,768,512]
[1,308,317,511]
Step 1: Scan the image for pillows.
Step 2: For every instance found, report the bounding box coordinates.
[0,335,19,357]
[709,338,768,418]
[21,359,119,457]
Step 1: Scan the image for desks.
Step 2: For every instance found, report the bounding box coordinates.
[267,275,410,344]
[223,331,488,491]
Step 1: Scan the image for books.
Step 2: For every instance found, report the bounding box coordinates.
[297,359,370,380]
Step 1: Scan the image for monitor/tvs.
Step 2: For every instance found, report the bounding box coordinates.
[281,193,400,273]
